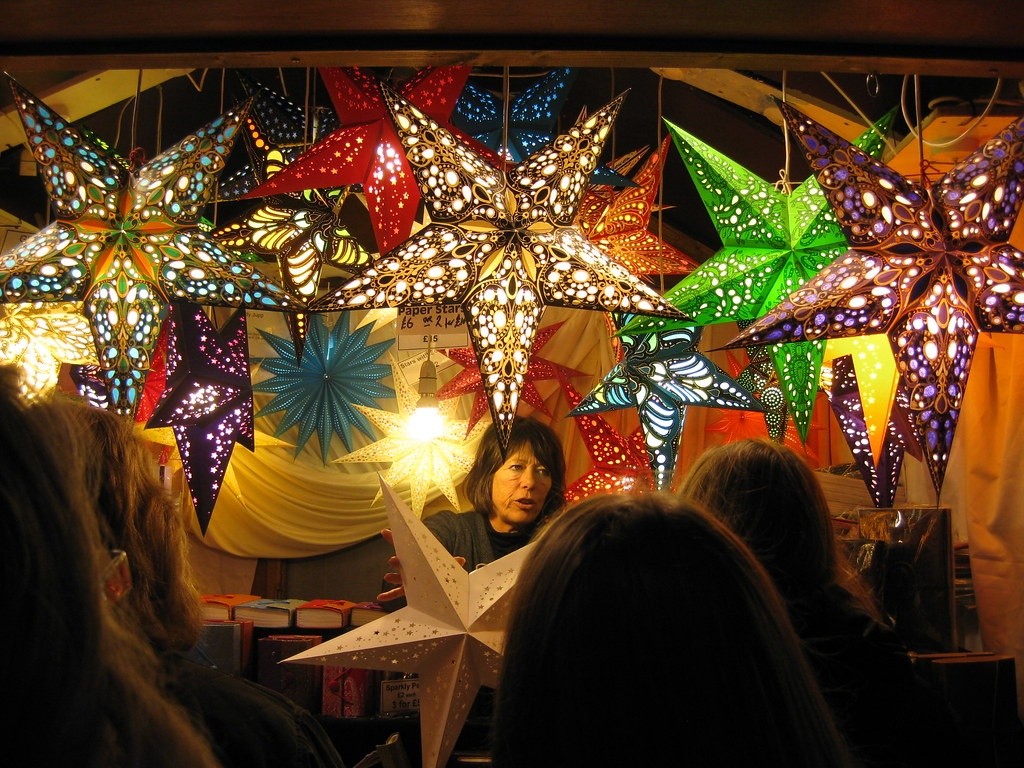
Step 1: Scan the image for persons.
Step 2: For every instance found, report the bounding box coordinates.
[675,437,936,767]
[491,485,849,767]
[2,376,219,768]
[378,412,570,768]
[27,395,345,768]
